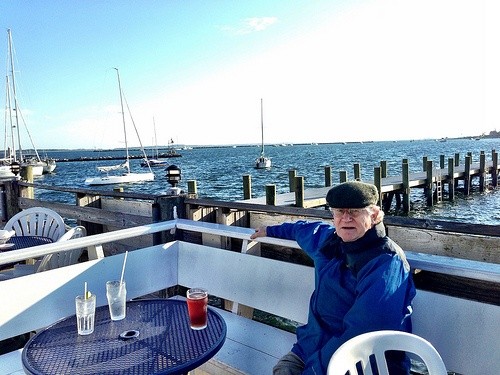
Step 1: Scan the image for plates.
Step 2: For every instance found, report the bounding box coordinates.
[0,243,15,250]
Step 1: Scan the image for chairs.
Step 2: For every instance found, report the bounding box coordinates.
[0,226,87,280]
[3,206,65,267]
[327,330,447,375]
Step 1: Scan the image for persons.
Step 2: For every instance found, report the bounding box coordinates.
[250,181,416,375]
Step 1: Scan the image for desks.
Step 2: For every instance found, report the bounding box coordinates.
[21,297,227,375]
[0,235,53,264]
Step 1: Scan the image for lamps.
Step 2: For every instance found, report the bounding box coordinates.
[163,165,182,188]
[9,162,21,176]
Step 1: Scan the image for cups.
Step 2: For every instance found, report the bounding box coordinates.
[106,280,127,320]
[187,288,208,330]
[75,295,96,335]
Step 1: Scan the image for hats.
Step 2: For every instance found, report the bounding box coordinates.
[326,182,379,208]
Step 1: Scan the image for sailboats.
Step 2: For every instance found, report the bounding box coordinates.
[0,26,57,182]
[85,67,156,186]
[254,97,275,169]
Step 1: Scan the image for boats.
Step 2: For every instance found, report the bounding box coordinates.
[139,157,168,169]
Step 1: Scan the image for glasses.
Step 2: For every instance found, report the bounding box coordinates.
[333,208,367,218]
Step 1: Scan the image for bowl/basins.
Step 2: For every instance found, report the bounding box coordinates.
[0,230,16,244]
